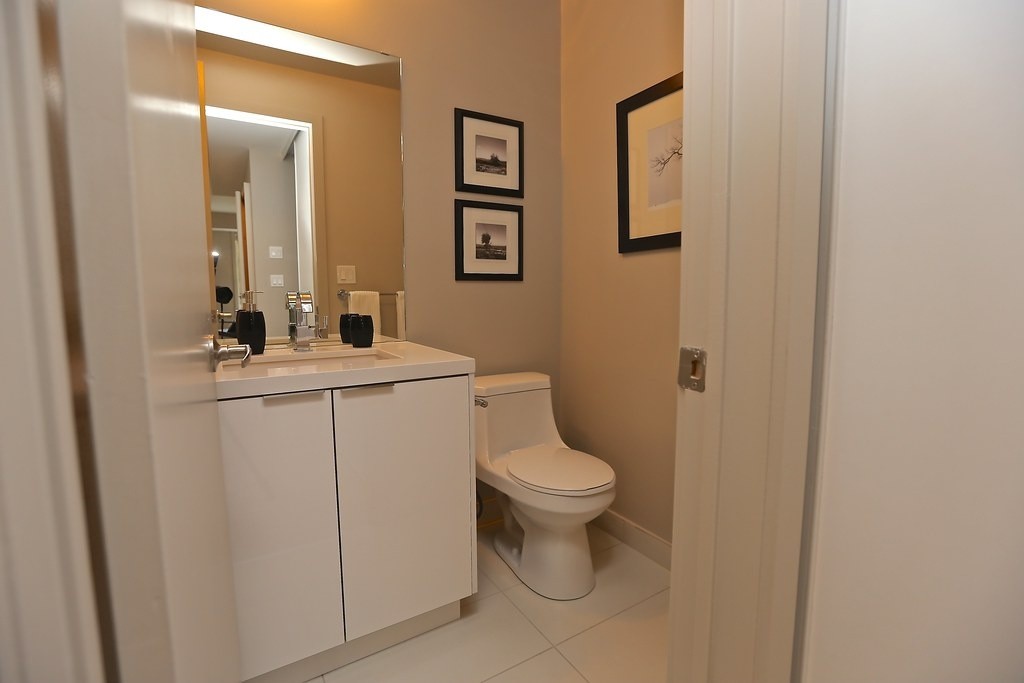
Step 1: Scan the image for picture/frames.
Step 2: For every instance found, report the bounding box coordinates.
[453,107,524,198]
[616,71,682,253]
[454,200,524,281]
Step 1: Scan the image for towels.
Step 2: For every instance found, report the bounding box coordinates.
[347,291,382,335]
[395,290,406,340]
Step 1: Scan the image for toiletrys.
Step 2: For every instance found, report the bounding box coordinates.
[235,289,267,356]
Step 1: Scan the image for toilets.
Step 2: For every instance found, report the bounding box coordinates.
[474,371,619,601]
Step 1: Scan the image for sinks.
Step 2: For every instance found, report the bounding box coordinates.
[218,333,353,351]
[216,344,405,401]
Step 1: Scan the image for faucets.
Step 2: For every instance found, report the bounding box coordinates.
[284,290,298,348]
[293,290,315,353]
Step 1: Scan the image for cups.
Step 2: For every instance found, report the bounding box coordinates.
[350,315,373,347]
[339,313,359,343]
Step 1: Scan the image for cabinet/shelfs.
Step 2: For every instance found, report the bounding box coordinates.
[218,374,477,683]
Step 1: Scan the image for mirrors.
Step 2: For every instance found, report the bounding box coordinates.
[195,4,406,350]
